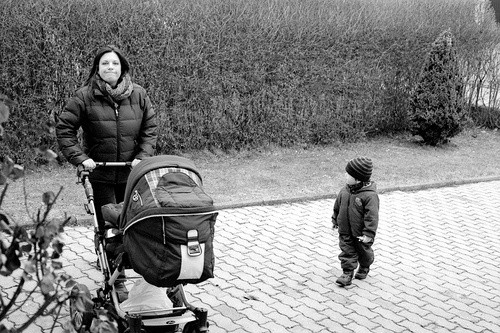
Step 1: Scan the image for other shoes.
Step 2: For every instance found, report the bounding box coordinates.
[113,283,129,302]
[355,269,367,280]
[336,274,353,284]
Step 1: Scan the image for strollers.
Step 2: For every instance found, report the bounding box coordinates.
[68,155,219,333]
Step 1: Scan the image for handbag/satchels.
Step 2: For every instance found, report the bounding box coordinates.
[118,279,174,315]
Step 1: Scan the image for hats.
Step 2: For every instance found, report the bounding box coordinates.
[345,157,372,181]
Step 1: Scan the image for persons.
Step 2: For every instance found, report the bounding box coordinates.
[331,157,380,287]
[55,46,159,302]
